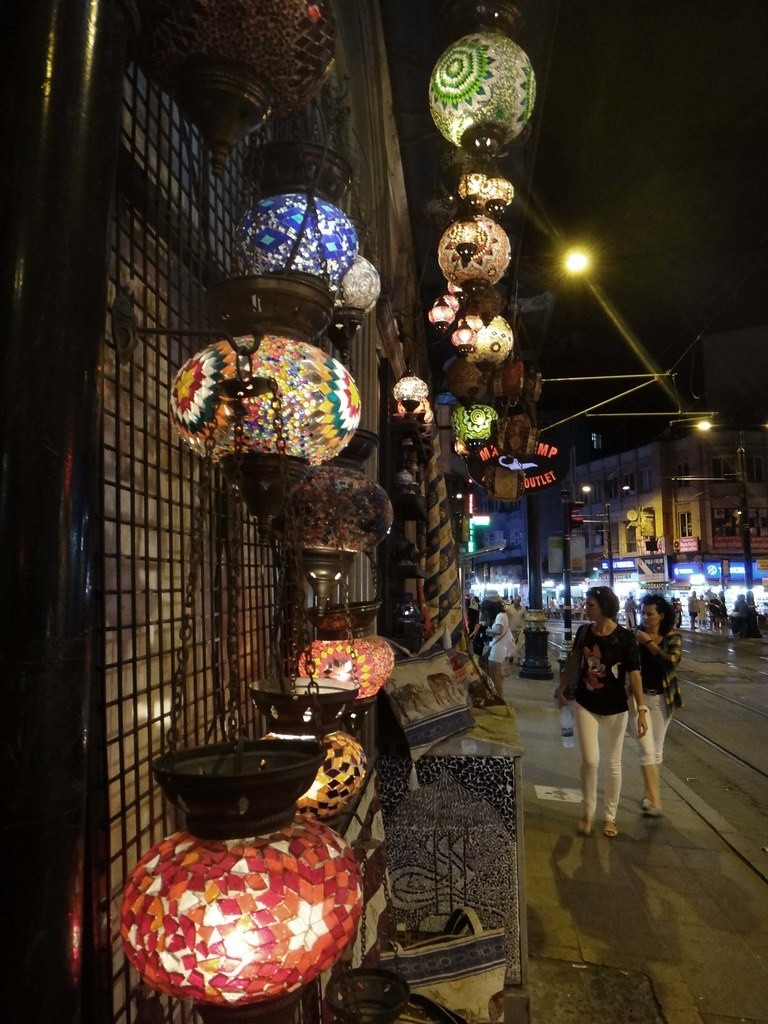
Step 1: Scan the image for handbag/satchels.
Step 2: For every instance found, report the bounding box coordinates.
[479,647,492,665]
[553,624,590,702]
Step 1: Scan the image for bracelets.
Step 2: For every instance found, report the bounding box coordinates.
[644,639,653,647]
[637,704,649,712]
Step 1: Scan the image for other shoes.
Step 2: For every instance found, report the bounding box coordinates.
[516,663,522,667]
[509,657,513,663]
[643,805,662,817]
[642,796,651,809]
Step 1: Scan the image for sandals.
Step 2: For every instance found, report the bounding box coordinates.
[578,817,593,835]
[601,818,617,837]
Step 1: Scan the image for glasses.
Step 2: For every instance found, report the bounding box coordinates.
[588,587,601,596]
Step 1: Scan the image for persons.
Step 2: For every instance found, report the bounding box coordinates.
[465,592,526,657]
[480,599,511,701]
[554,586,650,839]
[506,595,528,666]
[623,594,639,628]
[579,596,587,620]
[550,596,556,620]
[697,594,707,630]
[705,590,762,636]
[687,591,700,630]
[670,596,682,630]
[630,594,683,819]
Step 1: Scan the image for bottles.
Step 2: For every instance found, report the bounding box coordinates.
[560,704,574,747]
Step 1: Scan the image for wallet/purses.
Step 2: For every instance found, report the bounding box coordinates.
[479,633,492,641]
